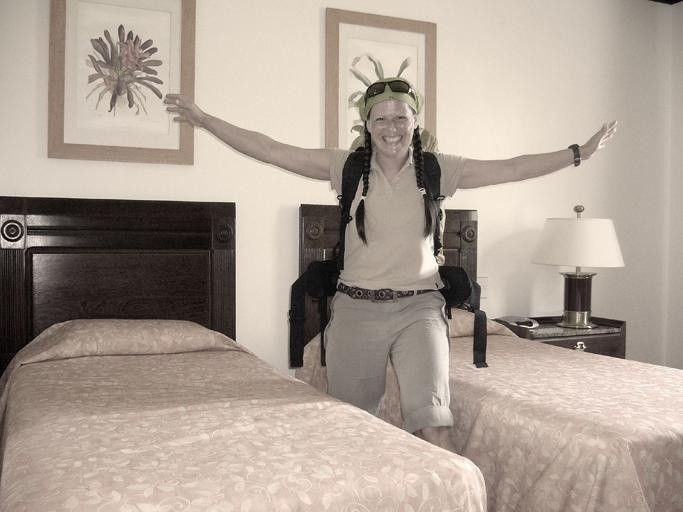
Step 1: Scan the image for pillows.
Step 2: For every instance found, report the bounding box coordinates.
[18,317,240,363]
[313,306,516,343]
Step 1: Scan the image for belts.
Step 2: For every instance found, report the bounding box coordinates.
[337,282,435,300]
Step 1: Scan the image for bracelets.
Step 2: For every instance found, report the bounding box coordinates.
[568,143,582,167]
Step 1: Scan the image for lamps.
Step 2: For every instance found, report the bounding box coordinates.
[527,203,627,328]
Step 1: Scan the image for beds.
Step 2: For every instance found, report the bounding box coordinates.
[297,202,683,511]
[1,194,492,511]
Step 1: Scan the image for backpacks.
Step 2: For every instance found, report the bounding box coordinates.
[287,125,488,368]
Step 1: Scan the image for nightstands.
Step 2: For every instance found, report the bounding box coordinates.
[491,315,628,360]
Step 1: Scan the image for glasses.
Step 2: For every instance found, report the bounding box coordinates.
[363,80,416,104]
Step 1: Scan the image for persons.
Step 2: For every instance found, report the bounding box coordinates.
[160,77,620,453]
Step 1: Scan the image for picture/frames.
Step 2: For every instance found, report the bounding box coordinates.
[323,5,440,155]
[46,0,198,168]
